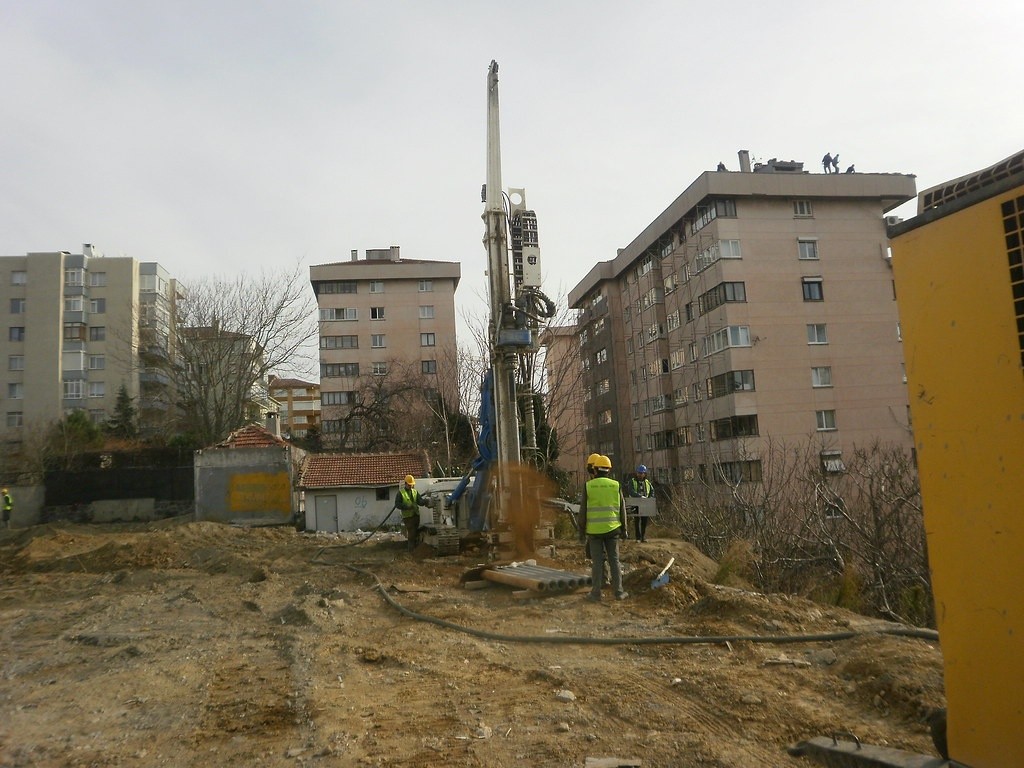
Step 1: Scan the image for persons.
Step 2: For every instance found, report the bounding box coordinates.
[586,453,610,589]
[578,456,629,602]
[822,153,855,174]
[0,489,14,528]
[628,465,655,543]
[395,475,429,553]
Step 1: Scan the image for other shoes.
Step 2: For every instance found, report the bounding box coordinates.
[615,592,629,601]
[584,592,601,603]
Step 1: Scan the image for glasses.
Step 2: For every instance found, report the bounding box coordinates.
[587,453,600,464]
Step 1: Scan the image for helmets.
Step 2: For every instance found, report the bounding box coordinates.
[405,475,416,486]
[637,465,647,474]
[594,456,612,469]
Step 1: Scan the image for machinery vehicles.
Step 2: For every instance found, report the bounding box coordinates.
[399,60,558,556]
